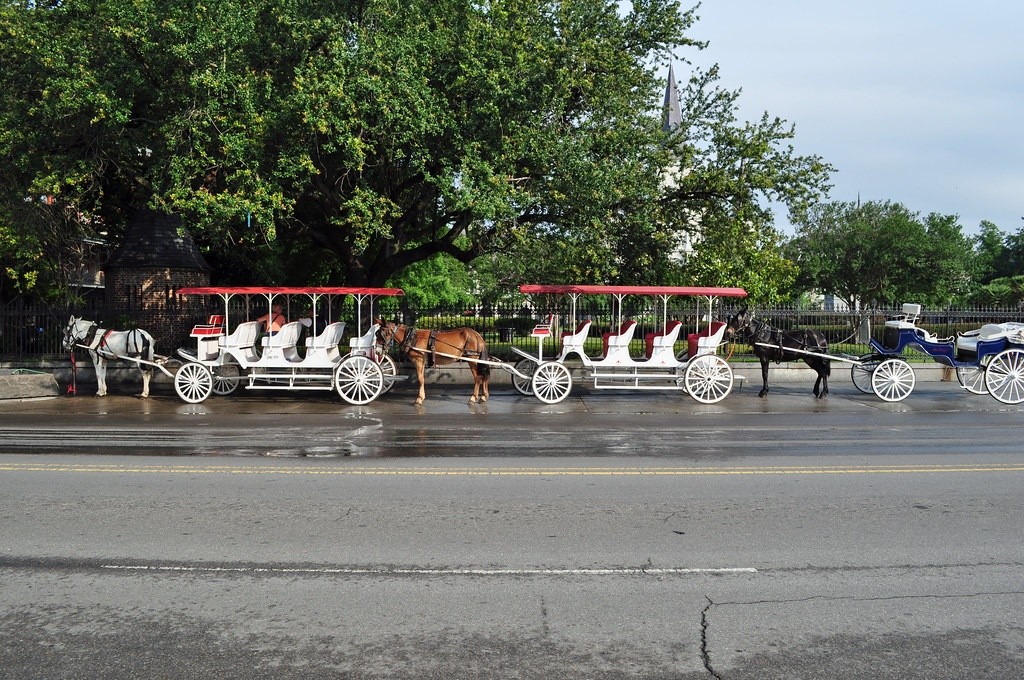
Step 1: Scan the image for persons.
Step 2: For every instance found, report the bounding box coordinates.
[257,305,285,337]
[298,303,327,338]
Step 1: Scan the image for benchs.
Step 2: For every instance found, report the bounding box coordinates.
[306,321,347,349]
[885,303,922,329]
[531,313,556,338]
[956,324,1005,351]
[916,326,955,346]
[349,323,382,348]
[688,321,728,347]
[262,321,304,347]
[190,315,228,338]
[645,320,682,347]
[218,321,261,346]
[603,320,637,346]
[560,318,592,346]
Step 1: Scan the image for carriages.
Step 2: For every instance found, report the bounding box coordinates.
[374,285,747,405]
[61,287,406,407]
[725,302,1024,404]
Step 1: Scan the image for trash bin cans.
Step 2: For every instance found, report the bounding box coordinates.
[499,327,516,343]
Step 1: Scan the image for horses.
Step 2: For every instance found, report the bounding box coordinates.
[374,316,490,409]
[723,304,830,400]
[62,315,155,399]
